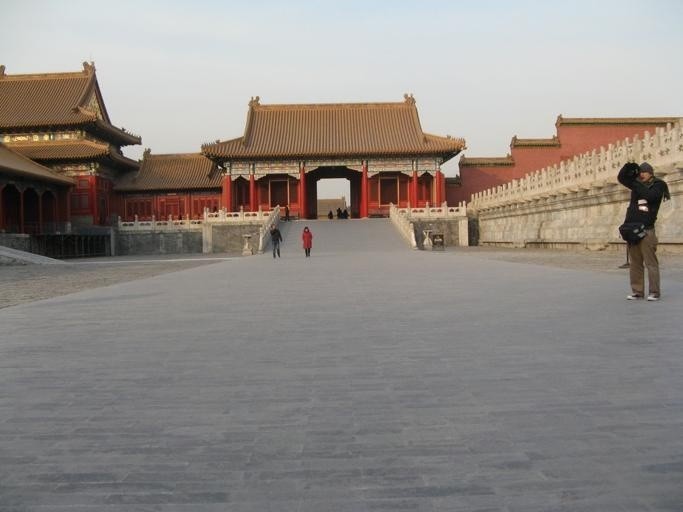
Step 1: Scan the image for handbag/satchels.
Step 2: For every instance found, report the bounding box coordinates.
[619,222,647,243]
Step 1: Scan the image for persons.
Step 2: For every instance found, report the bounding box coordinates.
[285,204,290,222]
[270,224,283,258]
[328,208,349,219]
[302,226,312,258]
[617,162,670,301]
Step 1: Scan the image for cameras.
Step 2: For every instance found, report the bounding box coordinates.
[627,166,640,178]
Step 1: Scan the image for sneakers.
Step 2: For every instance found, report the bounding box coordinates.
[627,294,642,300]
[647,294,661,302]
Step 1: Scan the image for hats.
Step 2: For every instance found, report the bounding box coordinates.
[640,162,653,174]
